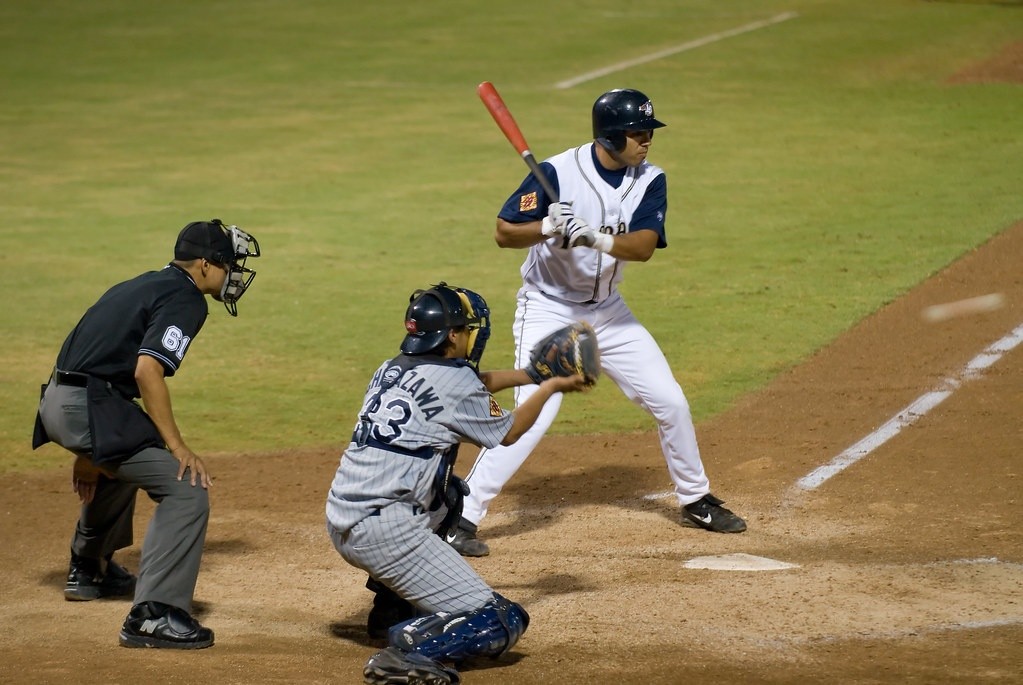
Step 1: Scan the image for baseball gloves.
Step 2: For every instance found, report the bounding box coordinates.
[520,322,600,394]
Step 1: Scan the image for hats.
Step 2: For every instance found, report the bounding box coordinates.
[174,218,234,261]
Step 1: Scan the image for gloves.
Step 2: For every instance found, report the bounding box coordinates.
[541,200,574,238]
[555,217,614,254]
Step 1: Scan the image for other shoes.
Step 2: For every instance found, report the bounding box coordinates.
[364,647,451,685]
[367,609,391,637]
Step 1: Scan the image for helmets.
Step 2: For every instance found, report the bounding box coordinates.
[399,281,469,354]
[592,88,667,153]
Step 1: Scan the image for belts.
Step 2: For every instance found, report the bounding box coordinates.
[56,371,89,388]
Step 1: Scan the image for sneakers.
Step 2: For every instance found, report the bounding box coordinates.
[64,547,137,601]
[118,600,214,649]
[681,493,747,533]
[443,516,490,558]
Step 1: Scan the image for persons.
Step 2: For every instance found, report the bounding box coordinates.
[325,281,586,685]
[442,88,748,556]
[31,219,260,650]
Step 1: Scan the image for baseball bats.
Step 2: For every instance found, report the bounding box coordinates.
[475,80,587,248]
